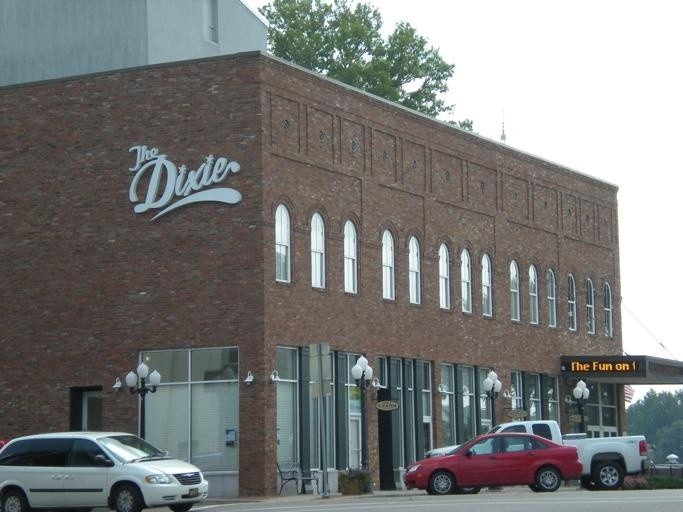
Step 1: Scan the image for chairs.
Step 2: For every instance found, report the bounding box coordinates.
[275,461,321,495]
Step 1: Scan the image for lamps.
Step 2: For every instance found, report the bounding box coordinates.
[371,377,380,390]
[269,369,280,385]
[244,371,254,386]
[112,376,122,391]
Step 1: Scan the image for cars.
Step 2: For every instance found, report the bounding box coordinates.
[403,433,583,495]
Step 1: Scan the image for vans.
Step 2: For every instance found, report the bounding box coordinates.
[0,431,209,511]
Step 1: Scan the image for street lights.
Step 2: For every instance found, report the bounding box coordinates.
[125,364,161,438]
[483,371,501,427]
[351,356,373,493]
[573,380,590,433]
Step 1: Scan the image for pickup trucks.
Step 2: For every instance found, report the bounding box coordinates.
[423,420,650,490]
